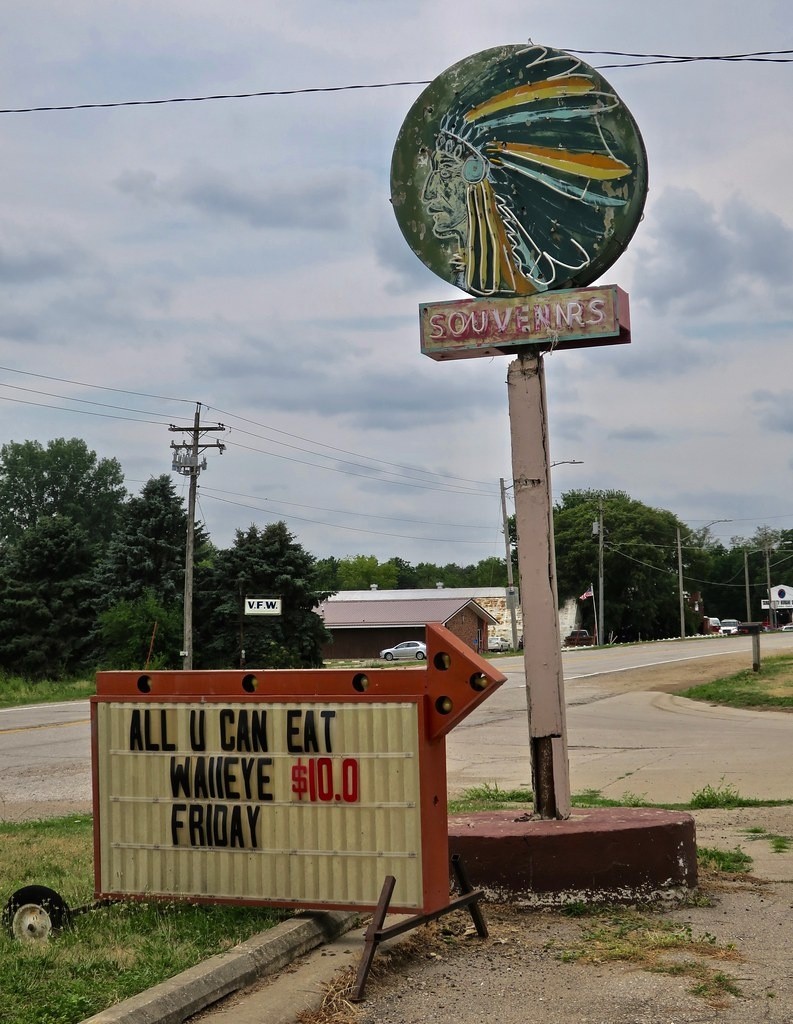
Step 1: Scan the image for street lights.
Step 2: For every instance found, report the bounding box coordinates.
[499,459,585,652]
[677,519,733,640]
[744,541,792,623]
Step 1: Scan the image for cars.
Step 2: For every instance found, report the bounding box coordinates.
[518,635,523,650]
[380,641,428,661]
[571,630,590,638]
[489,636,513,652]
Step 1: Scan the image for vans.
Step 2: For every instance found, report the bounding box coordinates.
[719,618,739,636]
